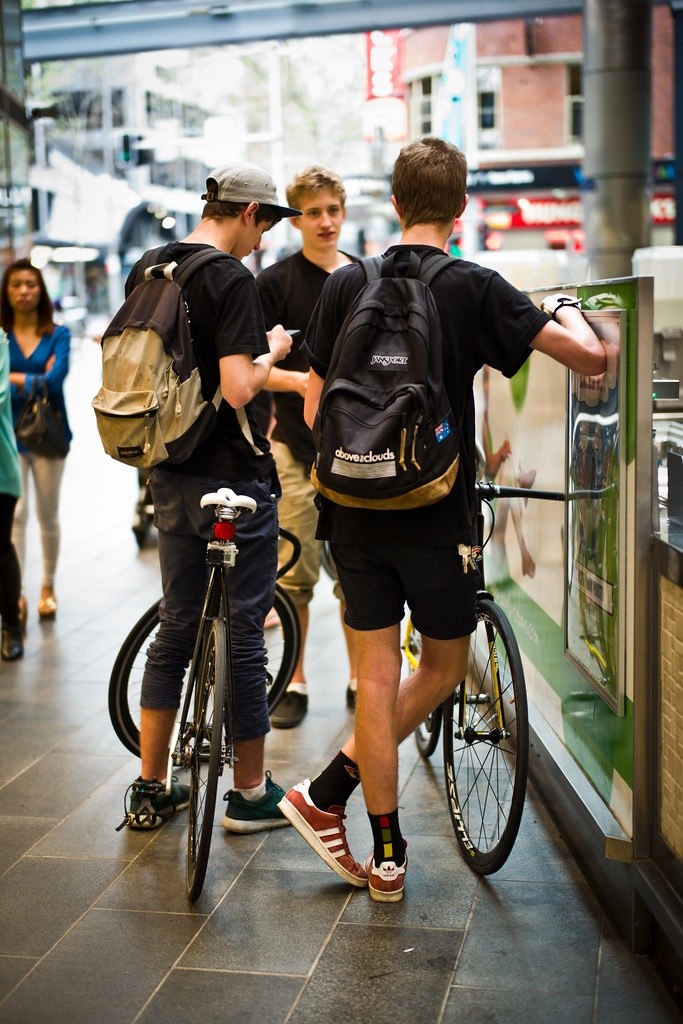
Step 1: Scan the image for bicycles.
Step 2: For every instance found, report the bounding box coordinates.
[106,486,303,904]
[400,481,616,874]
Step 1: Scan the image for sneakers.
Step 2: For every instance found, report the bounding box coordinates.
[359,850,408,900]
[275,780,368,887]
[220,770,290,832]
[126,786,191,829]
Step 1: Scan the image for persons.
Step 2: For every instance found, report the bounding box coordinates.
[273,140,606,908]
[0,333,25,661]
[122,160,302,835]
[258,169,374,731]
[1,258,74,628]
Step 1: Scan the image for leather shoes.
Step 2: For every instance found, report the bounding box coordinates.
[0,628,23,660]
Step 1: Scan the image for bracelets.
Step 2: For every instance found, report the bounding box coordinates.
[551,295,586,322]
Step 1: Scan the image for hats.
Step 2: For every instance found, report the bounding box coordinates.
[201,162,302,218]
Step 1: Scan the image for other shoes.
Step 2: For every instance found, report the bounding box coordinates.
[271,691,309,727]
[345,687,359,710]
[38,586,56,616]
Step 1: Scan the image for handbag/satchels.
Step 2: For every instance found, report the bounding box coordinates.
[20,373,70,458]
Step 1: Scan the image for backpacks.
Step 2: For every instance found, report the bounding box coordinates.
[91,244,239,469]
[309,248,459,512]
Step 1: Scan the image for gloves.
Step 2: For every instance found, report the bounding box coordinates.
[540,293,583,318]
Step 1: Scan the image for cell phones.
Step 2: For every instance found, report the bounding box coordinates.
[285,329,301,338]
